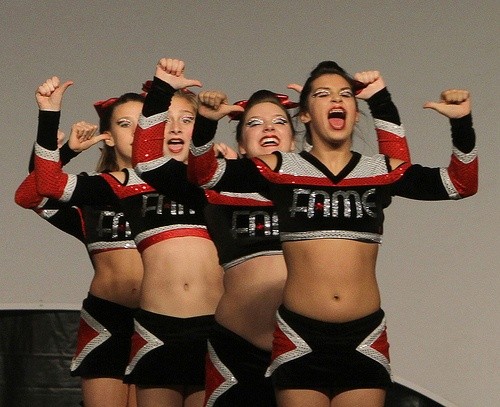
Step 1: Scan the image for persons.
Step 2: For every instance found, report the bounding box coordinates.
[33,76,303,407]
[188,60,478,407]
[130,59,410,407]
[14,93,238,407]
[26,128,85,243]
